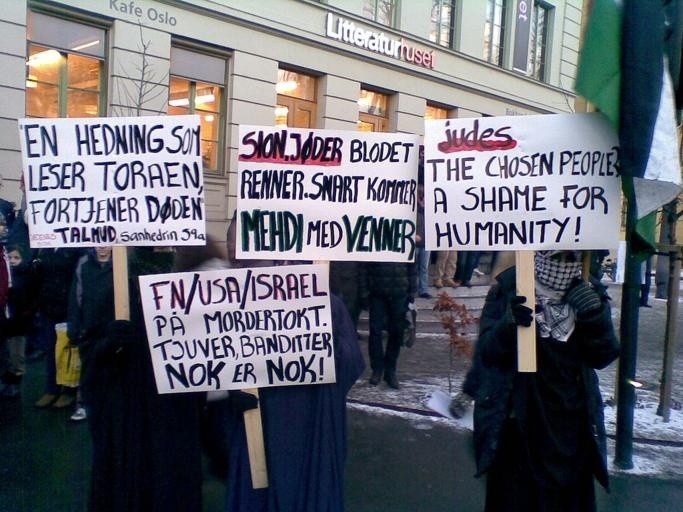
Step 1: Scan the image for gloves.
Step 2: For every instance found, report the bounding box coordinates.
[184,392,257,460]
[448,392,472,419]
[103,319,143,350]
[489,296,533,342]
[560,276,602,317]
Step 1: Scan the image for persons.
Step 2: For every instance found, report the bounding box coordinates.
[0,145,653,512]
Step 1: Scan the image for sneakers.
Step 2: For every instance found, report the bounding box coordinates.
[34,391,75,408]
[69,408,86,421]
[419,292,432,298]
[369,374,380,384]
[433,278,472,288]
[383,374,398,389]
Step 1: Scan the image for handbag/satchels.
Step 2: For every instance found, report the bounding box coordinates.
[400,297,417,348]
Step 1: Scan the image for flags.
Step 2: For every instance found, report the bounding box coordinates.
[574,0,683,255]
